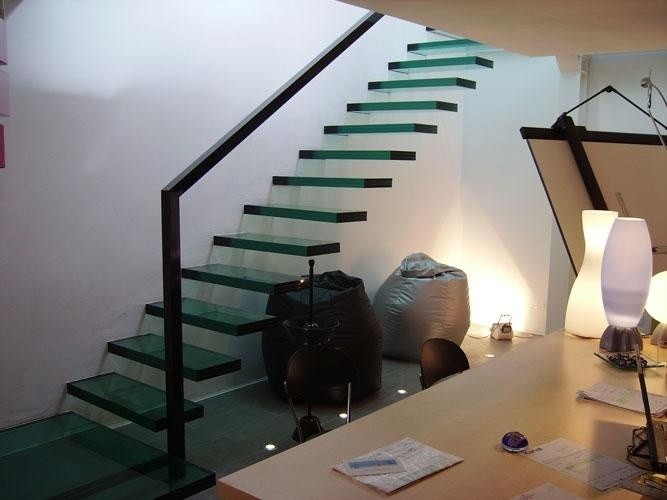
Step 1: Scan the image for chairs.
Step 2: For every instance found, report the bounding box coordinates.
[283,337,470,445]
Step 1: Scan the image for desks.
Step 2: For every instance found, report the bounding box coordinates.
[216,327,666,500]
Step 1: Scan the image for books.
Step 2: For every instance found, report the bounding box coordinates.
[574,378,667,419]
[332,434,466,495]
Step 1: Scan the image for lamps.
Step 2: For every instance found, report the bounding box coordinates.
[562,208,666,366]
